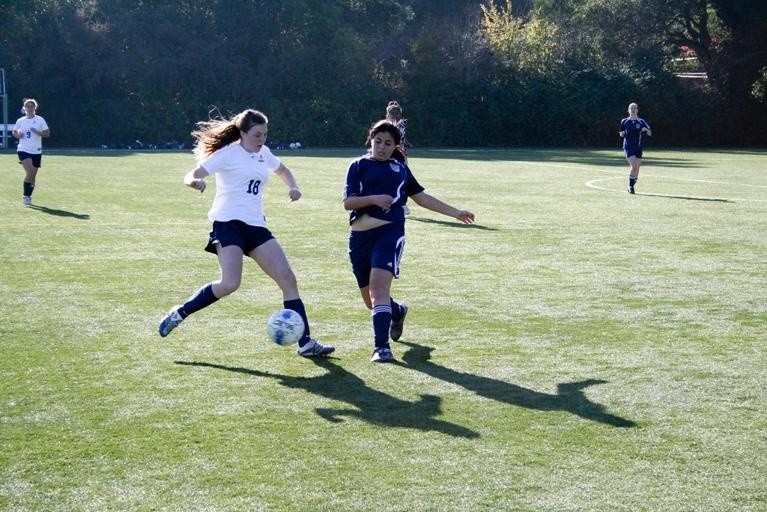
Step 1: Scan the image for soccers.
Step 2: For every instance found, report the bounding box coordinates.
[265,309,306,346]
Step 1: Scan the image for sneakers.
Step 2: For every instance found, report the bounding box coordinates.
[390,303,408,340]
[297,340,334,357]
[628,185,634,193]
[158,305,186,337]
[371,340,393,362]
[23,195,33,205]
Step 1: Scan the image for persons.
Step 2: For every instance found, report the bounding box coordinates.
[387,101,407,155]
[344,120,475,361]
[12,100,50,204]
[159,108,334,358]
[618,103,651,195]
